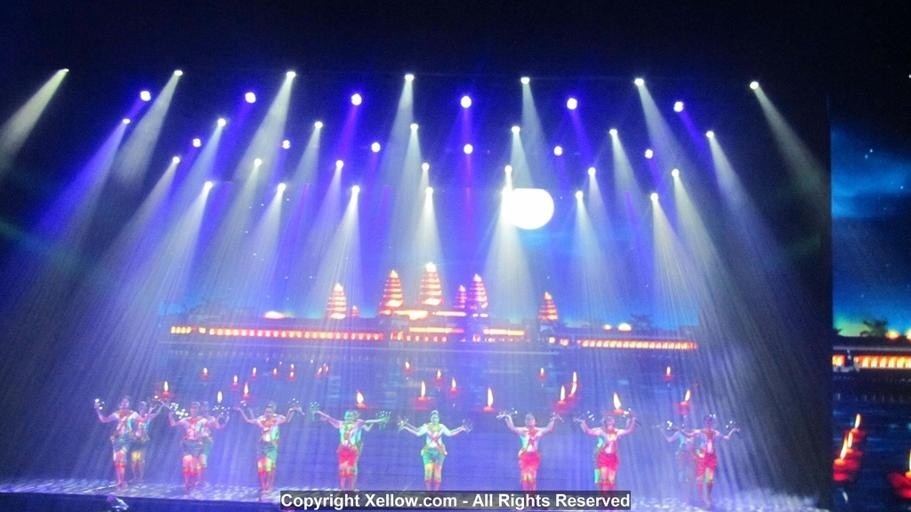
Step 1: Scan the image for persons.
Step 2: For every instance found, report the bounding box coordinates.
[93,396,739,509]
